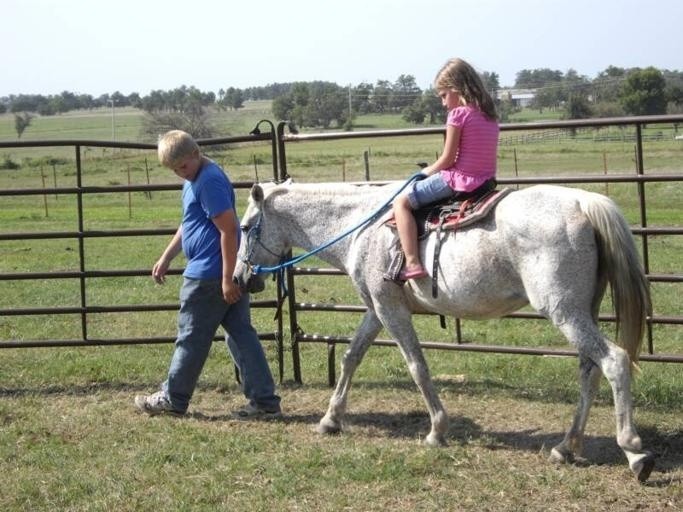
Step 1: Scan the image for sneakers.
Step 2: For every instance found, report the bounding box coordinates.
[230,394,281,419]
[135,391,188,417]
[397,263,427,280]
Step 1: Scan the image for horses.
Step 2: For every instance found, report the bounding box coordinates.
[232,178,656,484]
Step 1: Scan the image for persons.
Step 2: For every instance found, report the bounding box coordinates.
[132,128,287,422]
[391,57,503,281]
